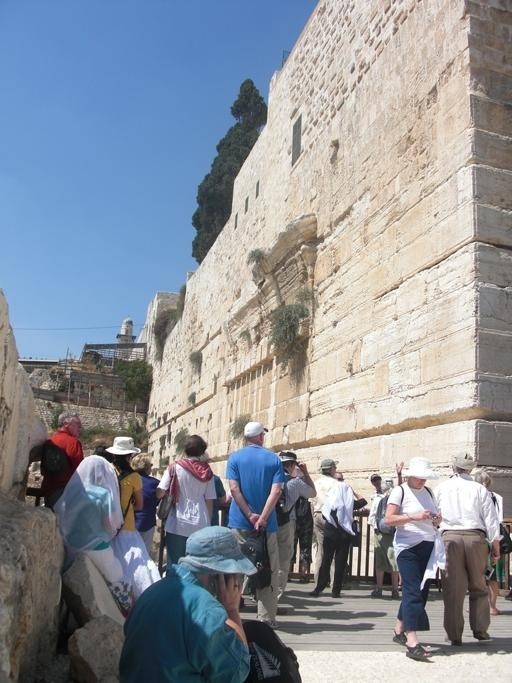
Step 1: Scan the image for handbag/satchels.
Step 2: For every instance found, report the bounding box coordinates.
[500,523,512,554]
[156,493,175,519]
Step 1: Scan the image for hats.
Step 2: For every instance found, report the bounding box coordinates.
[451,451,477,471]
[177,525,259,577]
[105,435,142,455]
[398,456,439,480]
[369,473,382,481]
[321,458,336,470]
[242,421,265,439]
[278,451,298,465]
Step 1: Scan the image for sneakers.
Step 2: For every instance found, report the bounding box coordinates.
[370,589,383,598]
[471,630,491,640]
[390,588,399,598]
[259,619,281,629]
[451,639,462,647]
[310,584,341,598]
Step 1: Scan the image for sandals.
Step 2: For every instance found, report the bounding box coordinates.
[405,643,433,660]
[392,628,408,644]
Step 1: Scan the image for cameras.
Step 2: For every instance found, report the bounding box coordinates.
[431,514,437,520]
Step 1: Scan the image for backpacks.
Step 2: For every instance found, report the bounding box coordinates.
[239,525,274,590]
[241,621,302,683]
[375,495,395,536]
